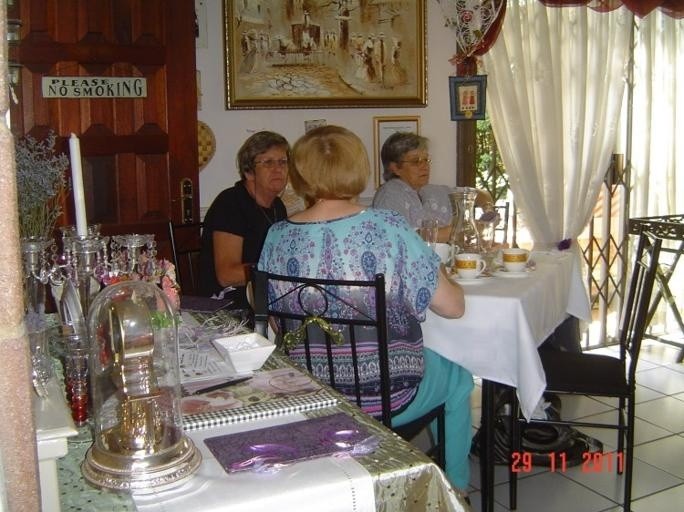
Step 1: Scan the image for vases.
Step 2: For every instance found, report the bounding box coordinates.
[19,239,56,384]
[15,129,70,239]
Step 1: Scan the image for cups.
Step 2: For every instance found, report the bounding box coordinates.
[394,157,433,167]
[252,157,288,169]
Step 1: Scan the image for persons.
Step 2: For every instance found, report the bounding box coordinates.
[252,125,473,505]
[193,131,290,314]
[371,131,502,247]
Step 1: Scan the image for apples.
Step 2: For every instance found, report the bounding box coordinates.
[67,132,88,237]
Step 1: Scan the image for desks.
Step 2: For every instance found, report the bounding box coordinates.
[35,309,436,511]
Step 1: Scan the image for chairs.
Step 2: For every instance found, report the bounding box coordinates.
[249,266,446,475]
[168,219,203,295]
[508,225,663,511]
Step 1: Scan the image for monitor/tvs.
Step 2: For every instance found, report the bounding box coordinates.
[168,364,335,432]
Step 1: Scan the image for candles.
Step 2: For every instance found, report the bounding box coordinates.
[67,132,88,237]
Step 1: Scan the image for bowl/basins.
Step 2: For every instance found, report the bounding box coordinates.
[256,200,278,227]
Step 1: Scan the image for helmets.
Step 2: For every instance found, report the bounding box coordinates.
[491,268,531,279]
[451,274,491,286]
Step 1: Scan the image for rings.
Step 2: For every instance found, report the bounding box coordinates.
[196,377,252,394]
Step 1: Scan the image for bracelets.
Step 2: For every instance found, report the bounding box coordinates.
[211,332,278,375]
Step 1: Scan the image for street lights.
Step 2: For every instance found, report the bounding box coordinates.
[470,393,603,469]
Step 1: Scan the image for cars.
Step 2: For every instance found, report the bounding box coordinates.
[430,241,460,268]
[475,221,495,255]
[418,218,439,251]
[454,252,488,279]
[502,248,531,272]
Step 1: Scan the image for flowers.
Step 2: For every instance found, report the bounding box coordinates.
[15,129,70,239]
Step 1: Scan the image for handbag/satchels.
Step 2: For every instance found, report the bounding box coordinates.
[481,202,493,208]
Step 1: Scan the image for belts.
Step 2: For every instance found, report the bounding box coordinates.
[49,333,106,443]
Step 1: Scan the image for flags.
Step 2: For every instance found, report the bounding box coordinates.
[19,239,56,384]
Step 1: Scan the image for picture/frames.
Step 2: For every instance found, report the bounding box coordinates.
[373,115,422,189]
[448,74,487,121]
[35,309,436,511]
[223,2,428,108]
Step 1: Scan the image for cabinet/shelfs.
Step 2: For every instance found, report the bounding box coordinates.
[628,214,684,363]
[449,190,479,256]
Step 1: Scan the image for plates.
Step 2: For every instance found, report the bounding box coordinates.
[628,214,684,363]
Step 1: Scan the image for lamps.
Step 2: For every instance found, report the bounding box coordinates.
[249,266,446,475]
[168,219,203,295]
[508,225,663,511]
